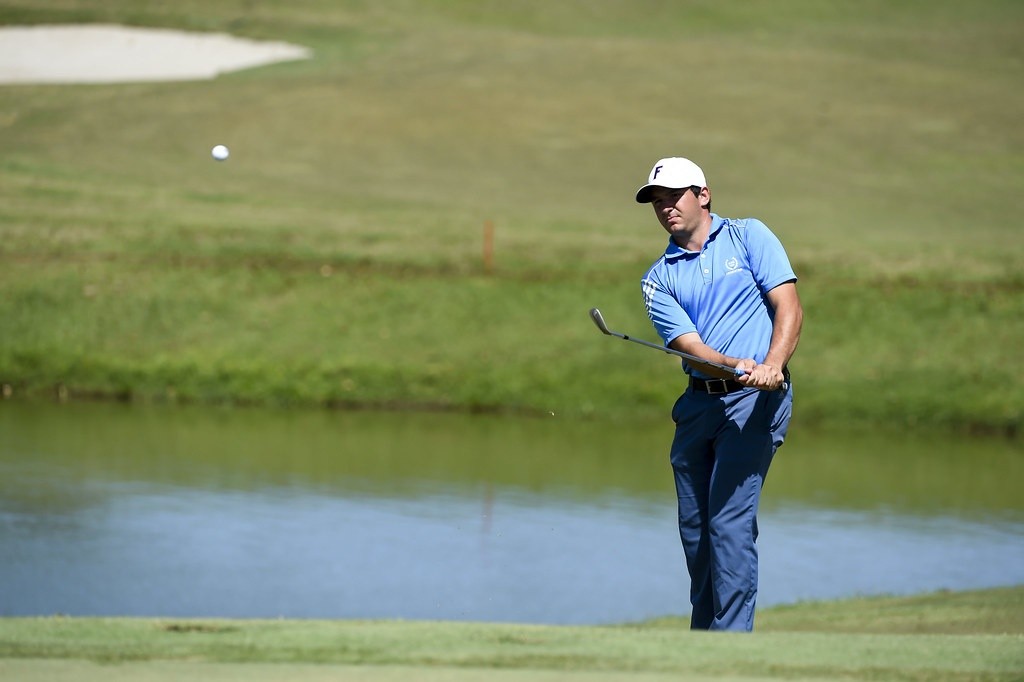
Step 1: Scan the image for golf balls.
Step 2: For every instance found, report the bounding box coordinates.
[212,144,229,160]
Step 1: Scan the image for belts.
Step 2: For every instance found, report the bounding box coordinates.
[689,368,787,394]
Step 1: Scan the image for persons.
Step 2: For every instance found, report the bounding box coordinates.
[635,156,804,630]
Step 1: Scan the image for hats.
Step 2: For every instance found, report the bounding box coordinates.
[636,157,706,203]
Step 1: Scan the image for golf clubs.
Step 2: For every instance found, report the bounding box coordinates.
[590,305,746,376]
